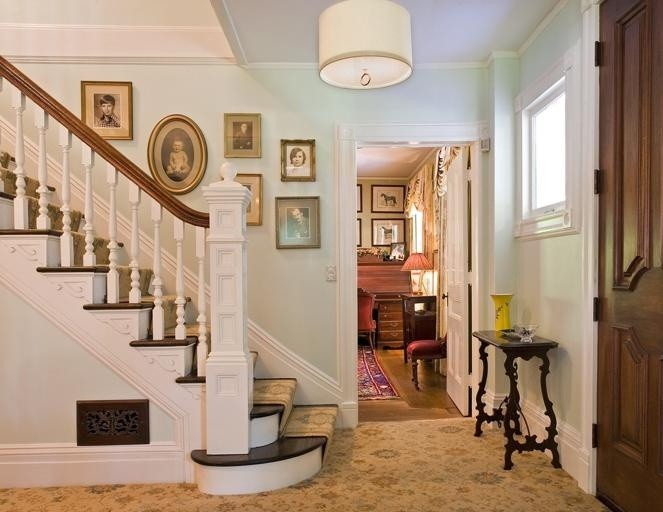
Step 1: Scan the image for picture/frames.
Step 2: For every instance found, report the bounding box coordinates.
[356,218,362,248]
[222,111,261,160]
[278,138,317,183]
[146,113,209,196]
[356,183,363,213]
[78,80,134,141]
[220,173,262,226]
[272,194,322,250]
[387,241,405,261]
[369,183,406,214]
[370,218,406,247]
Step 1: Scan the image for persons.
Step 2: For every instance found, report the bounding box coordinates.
[95,95,121,127]
[233,123,252,150]
[288,209,309,237]
[286,148,310,176]
[165,139,190,175]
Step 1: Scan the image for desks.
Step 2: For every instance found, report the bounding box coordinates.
[357,248,411,352]
[470,328,561,471]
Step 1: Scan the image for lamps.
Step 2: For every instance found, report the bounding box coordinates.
[316,0,413,93]
[399,252,432,295]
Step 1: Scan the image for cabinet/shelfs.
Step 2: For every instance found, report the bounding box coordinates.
[401,292,436,364]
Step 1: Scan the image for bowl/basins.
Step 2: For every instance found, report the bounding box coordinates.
[512,323,538,344]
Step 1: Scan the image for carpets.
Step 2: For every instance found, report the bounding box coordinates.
[357,344,401,401]
[0,418,614,511]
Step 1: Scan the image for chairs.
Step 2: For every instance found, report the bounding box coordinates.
[357,287,380,352]
[404,332,446,391]
